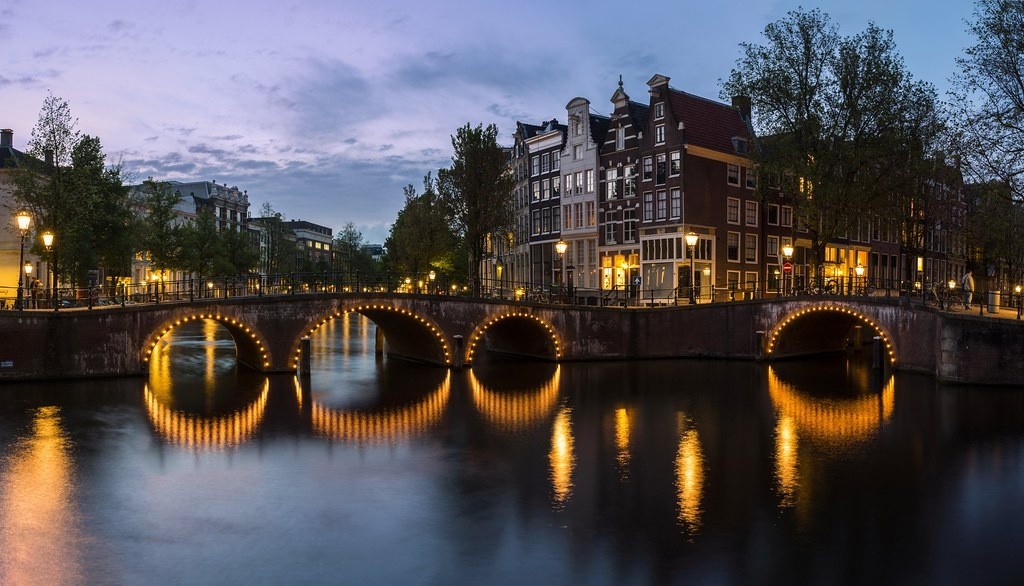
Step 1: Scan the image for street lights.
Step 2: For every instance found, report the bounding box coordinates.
[1016,286,1022,318]
[429,271,437,294]
[405,277,410,293]
[17,211,30,310]
[23,262,32,308]
[783,244,793,297]
[42,231,54,307]
[703,268,711,299]
[949,279,956,304]
[685,231,700,303]
[141,280,146,302]
[555,240,567,304]
[854,264,863,295]
[208,281,213,297]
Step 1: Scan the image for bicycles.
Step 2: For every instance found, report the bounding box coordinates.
[921,281,964,312]
[808,276,839,294]
[849,280,877,297]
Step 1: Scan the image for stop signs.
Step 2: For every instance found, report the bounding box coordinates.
[784,262,792,273]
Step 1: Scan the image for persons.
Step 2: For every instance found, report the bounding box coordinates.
[961,270,974,310]
[30,276,37,309]
[33,280,43,307]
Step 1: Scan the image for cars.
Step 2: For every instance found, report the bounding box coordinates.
[25,294,72,308]
